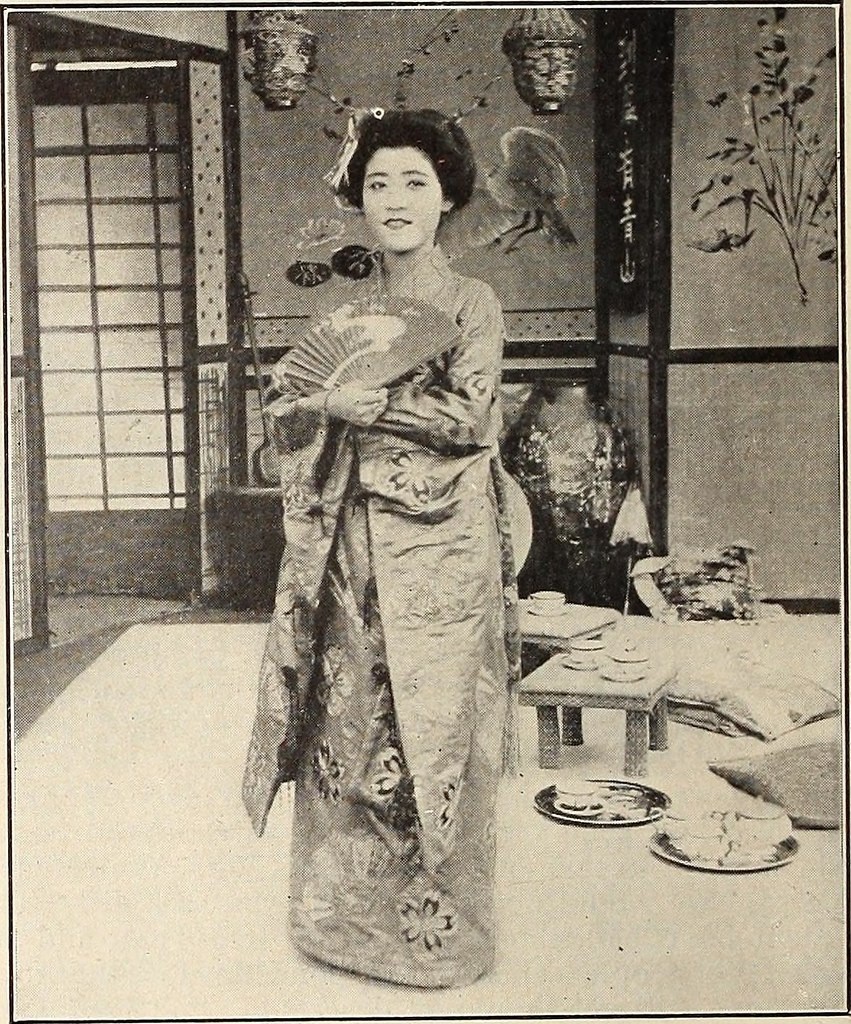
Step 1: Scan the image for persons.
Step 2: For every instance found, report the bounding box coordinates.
[240,106,508,986]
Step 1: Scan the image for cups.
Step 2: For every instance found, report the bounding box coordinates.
[605,645,649,674]
[569,640,606,662]
[528,591,566,609]
[554,783,602,806]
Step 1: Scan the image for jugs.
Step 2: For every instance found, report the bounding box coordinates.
[680,826,728,865]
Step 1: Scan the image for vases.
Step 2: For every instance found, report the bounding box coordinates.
[502,378,642,620]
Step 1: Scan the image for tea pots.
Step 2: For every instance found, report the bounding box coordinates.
[649,798,709,848]
[711,795,794,850]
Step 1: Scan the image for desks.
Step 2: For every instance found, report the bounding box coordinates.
[516,600,621,664]
[519,652,681,777]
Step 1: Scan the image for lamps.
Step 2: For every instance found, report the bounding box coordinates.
[248,10,320,111]
[502,8,587,115]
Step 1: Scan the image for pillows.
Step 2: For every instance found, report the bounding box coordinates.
[631,540,762,625]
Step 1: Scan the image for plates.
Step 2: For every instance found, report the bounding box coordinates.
[527,603,568,617]
[553,798,609,817]
[562,657,599,671]
[602,671,647,682]
